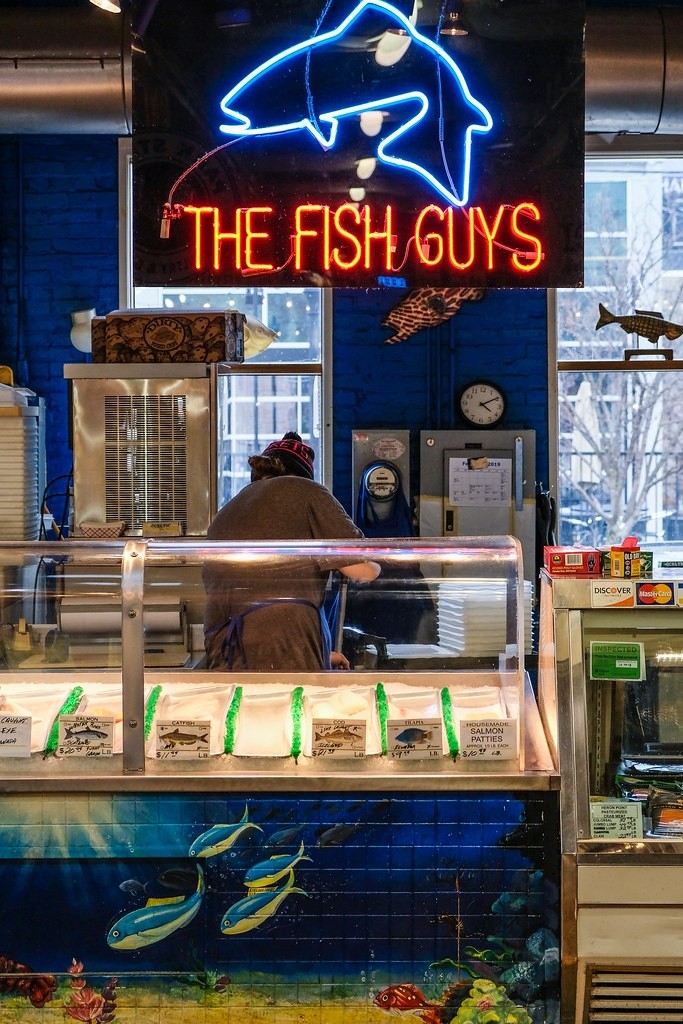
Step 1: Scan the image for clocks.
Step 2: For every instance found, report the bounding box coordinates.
[455,379,508,431]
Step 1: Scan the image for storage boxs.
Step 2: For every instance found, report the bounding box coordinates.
[91,309,248,364]
[543,544,653,580]
[142,520,183,538]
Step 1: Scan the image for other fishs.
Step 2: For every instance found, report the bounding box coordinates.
[314,728,364,747]
[63,726,109,744]
[394,727,434,745]
[595,303,683,345]
[157,728,209,749]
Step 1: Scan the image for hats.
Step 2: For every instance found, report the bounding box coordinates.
[250,432,314,481]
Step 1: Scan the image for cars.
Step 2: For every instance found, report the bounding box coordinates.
[556,483,683,555]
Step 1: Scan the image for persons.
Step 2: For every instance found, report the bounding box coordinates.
[203,432,382,672]
